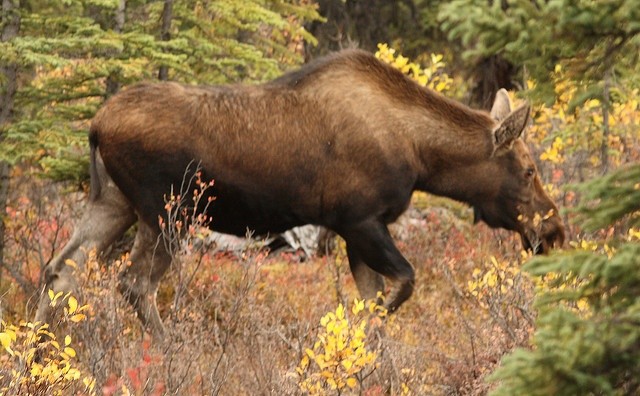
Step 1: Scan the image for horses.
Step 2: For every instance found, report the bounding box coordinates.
[20,50,565,367]
